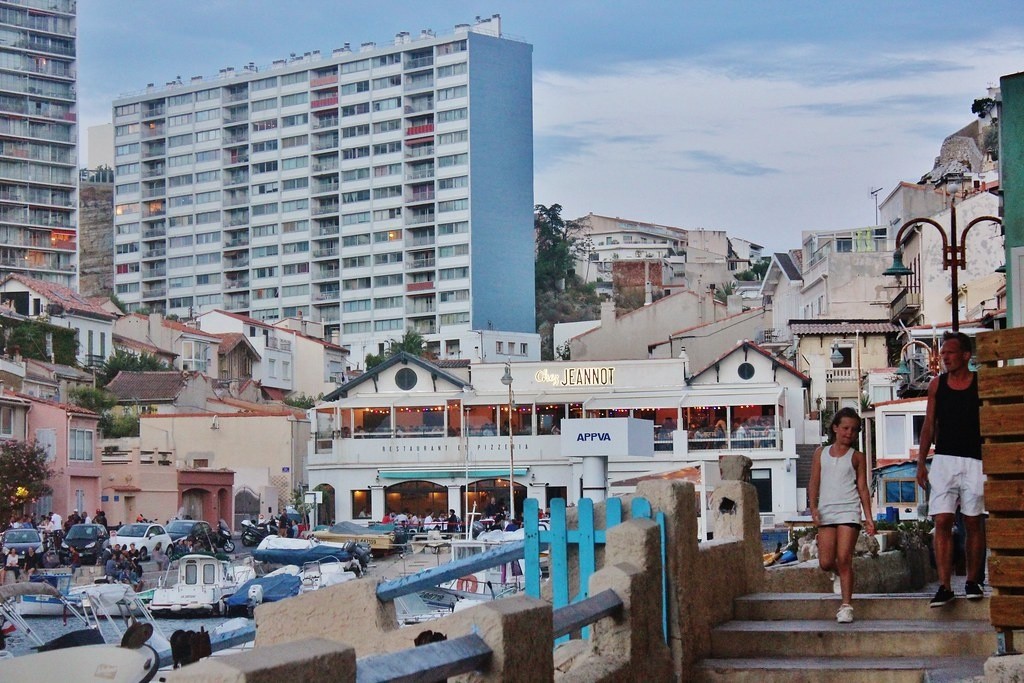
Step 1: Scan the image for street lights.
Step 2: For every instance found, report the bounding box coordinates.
[894,321,978,377]
[882,184,1006,332]
[500,362,515,519]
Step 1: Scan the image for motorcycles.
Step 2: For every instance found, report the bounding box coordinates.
[212,518,235,553]
[241,520,279,547]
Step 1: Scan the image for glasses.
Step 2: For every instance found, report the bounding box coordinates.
[939,351,964,355]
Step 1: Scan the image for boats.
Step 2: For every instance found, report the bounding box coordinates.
[301,521,396,550]
[0,583,255,683]
[414,519,549,609]
[0,554,363,617]
[253,534,372,569]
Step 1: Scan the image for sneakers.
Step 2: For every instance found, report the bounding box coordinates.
[831,573,841,595]
[930,585,954,607]
[836,604,854,623]
[965,580,984,599]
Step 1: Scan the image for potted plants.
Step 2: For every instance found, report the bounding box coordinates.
[874,517,936,554]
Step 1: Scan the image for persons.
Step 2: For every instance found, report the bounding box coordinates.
[278,508,288,538]
[915,331,988,608]
[286,522,294,538]
[338,410,820,561]
[24,546,38,582]
[1,508,108,540]
[808,407,876,623]
[68,545,81,573]
[292,519,299,538]
[4,547,25,583]
[99,535,210,589]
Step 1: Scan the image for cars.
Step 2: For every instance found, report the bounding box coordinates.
[103,523,174,562]
[0,529,43,567]
[59,524,109,565]
[164,519,216,552]
